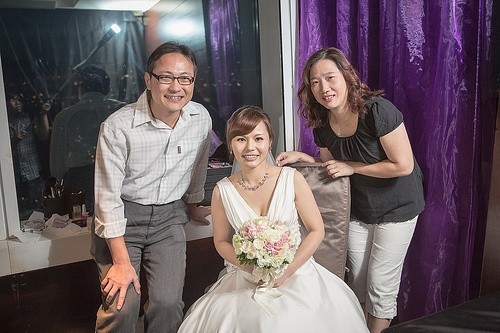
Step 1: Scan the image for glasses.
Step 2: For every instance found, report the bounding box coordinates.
[148,70,194,85]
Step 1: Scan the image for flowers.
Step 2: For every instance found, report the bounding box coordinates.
[233,216,298,268]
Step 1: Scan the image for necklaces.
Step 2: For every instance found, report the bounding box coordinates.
[336,114,356,136]
[238,169,270,190]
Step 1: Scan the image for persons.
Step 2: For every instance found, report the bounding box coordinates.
[46,64,127,214]
[175,104,370,333]
[274,47,426,333]
[89,40,213,333]
[7,90,51,219]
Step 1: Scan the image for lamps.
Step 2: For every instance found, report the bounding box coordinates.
[53,24,120,101]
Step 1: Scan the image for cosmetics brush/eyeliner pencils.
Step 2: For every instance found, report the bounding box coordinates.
[42,176,64,198]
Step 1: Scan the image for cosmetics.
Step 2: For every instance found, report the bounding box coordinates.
[87,217,94,229]
[82,204,88,220]
[72,204,81,219]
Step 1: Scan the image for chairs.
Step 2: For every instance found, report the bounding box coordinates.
[284,162,351,278]
